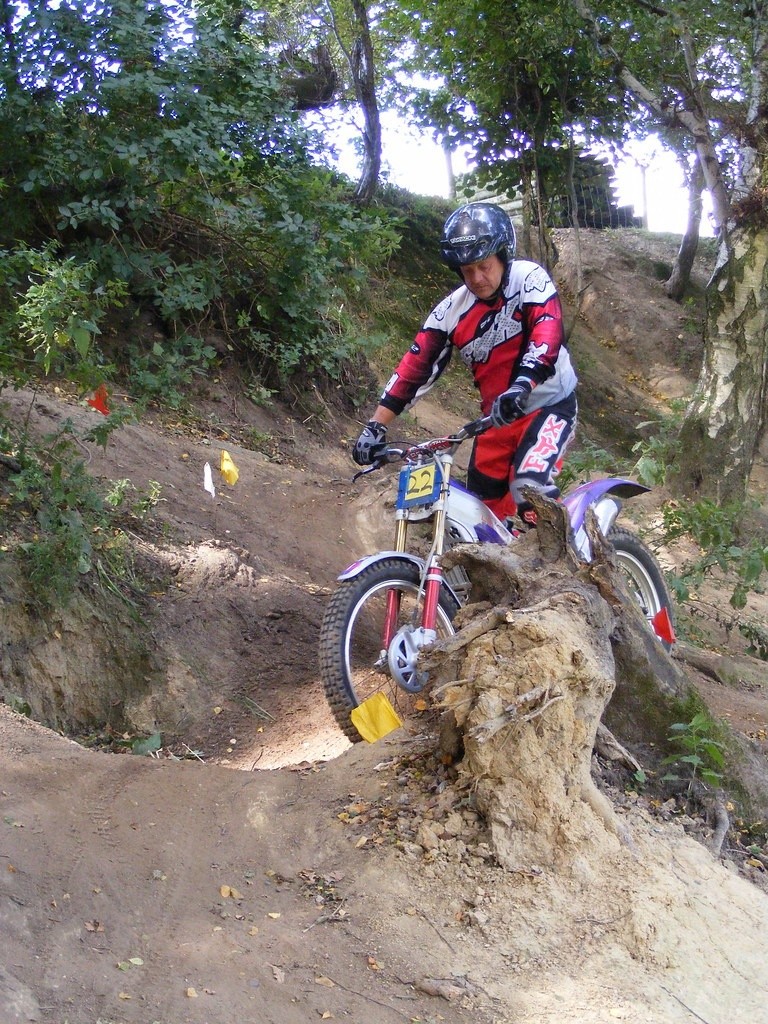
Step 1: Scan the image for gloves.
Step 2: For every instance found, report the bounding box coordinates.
[352,419,388,466]
[490,380,532,429]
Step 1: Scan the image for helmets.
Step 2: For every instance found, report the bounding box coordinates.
[440,202,516,279]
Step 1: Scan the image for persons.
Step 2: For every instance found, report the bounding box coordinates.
[353,203,579,539]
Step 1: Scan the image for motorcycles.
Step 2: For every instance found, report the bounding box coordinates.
[317,397,674,745]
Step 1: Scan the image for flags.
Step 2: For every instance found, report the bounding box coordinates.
[204,450,240,498]
[349,690,403,744]
[651,607,675,644]
[87,383,113,416]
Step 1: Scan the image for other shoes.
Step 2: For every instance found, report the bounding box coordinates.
[511,529,520,539]
